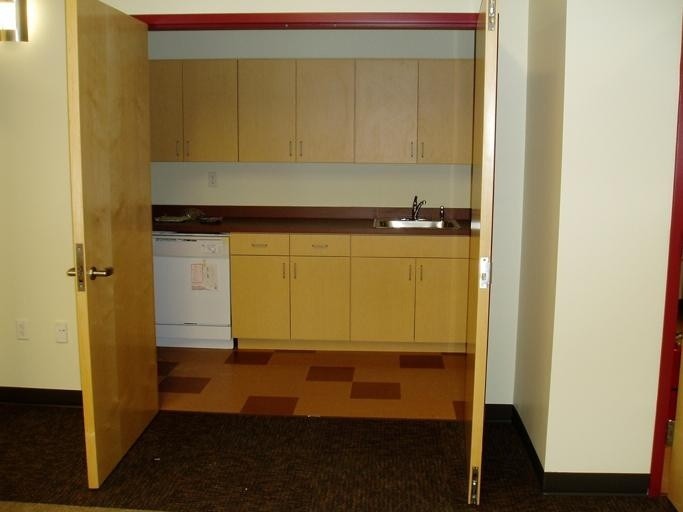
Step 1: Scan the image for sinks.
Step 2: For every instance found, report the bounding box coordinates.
[372,216,460,230]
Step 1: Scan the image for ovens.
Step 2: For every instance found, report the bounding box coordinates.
[152,237,234,350]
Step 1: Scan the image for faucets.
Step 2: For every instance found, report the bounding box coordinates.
[412,194,427,219]
[439,205,445,220]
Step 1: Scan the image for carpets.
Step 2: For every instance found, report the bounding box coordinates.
[1,403,680,512]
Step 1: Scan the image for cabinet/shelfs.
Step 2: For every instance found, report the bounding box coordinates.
[355,57,474,165]
[230,234,349,340]
[238,57,354,162]
[350,256,472,346]
[141,58,238,162]
